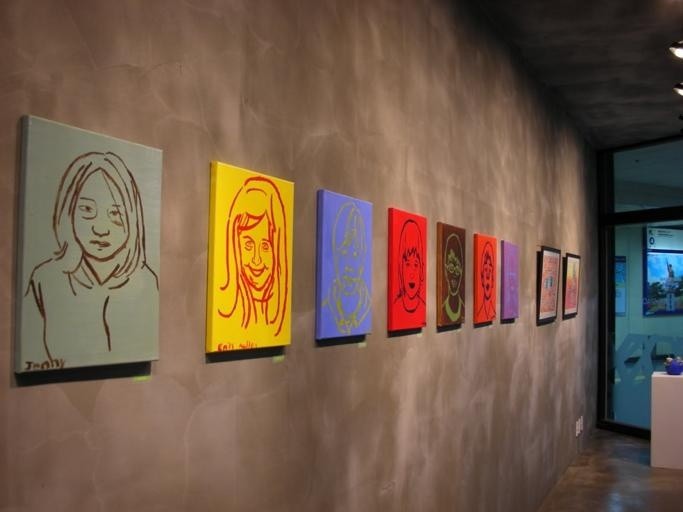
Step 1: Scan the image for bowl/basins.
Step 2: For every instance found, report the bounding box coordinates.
[666,362,682,374]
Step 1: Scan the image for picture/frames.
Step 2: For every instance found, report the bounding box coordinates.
[536,246,561,325]
[563,253,581,319]
[614,255,627,316]
